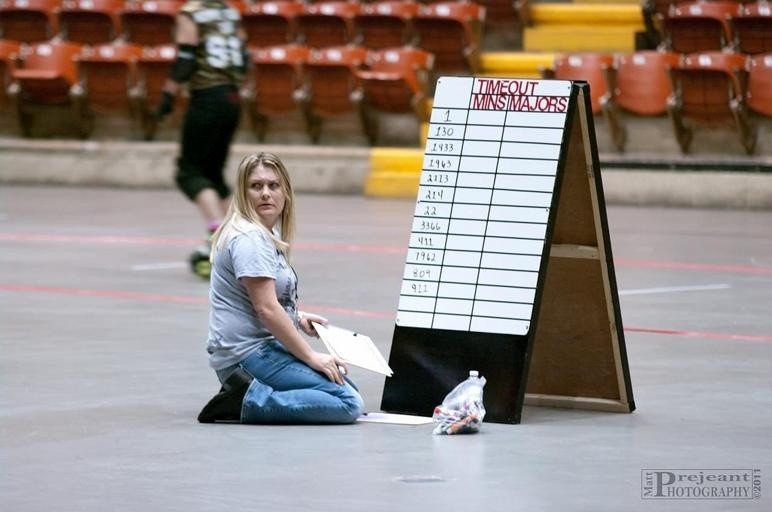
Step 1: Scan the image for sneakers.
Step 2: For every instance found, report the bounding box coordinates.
[197,368,254,424]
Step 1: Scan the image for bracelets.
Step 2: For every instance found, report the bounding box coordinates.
[296,308,306,322]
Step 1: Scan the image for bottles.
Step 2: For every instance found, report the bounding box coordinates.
[465,370,483,401]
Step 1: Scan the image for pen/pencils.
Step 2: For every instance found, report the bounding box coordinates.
[435,401,481,434]
[339,366,347,376]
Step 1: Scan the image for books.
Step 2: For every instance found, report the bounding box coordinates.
[310,317,394,380]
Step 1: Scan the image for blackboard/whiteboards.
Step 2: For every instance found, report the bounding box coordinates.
[380,75,573,424]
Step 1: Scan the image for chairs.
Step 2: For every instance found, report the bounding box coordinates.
[0,0,768,154]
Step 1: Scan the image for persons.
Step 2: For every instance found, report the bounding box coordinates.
[146,0,255,282]
[196,147,367,427]
